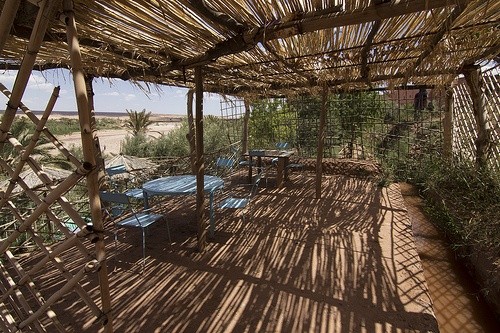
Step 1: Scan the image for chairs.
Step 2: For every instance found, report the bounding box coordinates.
[100,190,170,272]
[211,143,290,200]
[106,165,163,214]
[218,172,261,244]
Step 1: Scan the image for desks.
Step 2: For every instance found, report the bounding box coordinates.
[142,174,224,241]
[241,150,295,187]
